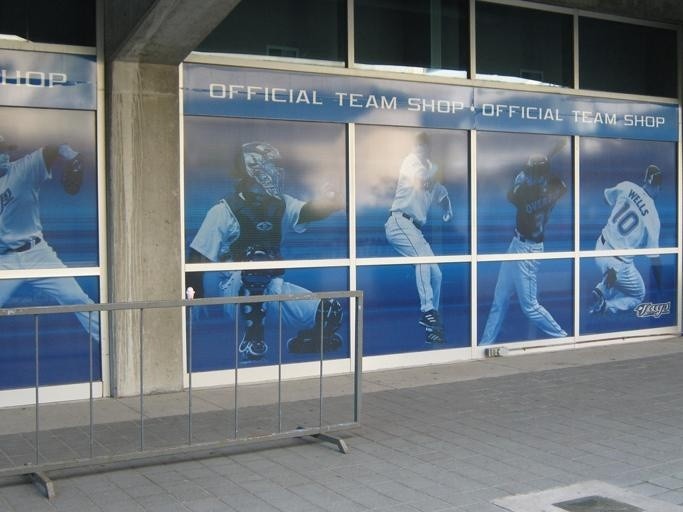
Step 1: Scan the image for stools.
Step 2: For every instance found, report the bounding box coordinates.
[6,236,41,253]
[514,231,544,243]
[601,235,627,264]
[391,211,423,230]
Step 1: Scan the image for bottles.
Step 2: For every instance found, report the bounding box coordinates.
[643,165,663,185]
[522,154,550,176]
[0,134,18,153]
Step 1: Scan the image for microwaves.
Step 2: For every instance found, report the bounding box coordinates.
[523,138,567,176]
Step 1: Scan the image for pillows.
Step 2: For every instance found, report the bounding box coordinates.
[234,140,286,202]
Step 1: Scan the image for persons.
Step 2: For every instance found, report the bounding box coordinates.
[184,139,349,366]
[0,134,100,346]
[383,129,455,345]
[587,163,662,315]
[479,153,568,346]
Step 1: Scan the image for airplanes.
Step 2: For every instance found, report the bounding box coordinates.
[60,153,85,195]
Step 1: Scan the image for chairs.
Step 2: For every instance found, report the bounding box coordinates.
[419,309,446,329]
[286,334,344,354]
[424,331,447,344]
[588,283,612,314]
[241,336,268,360]
